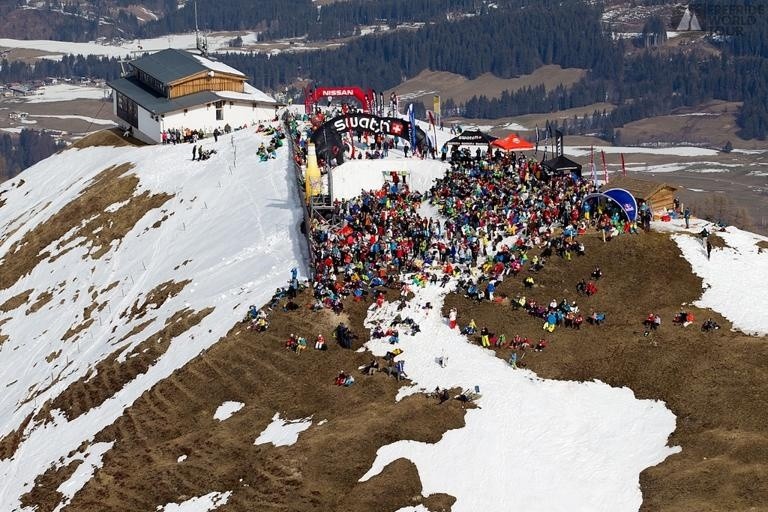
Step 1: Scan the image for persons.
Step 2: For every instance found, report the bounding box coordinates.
[439,389,449,404]
[288,97,294,105]
[161,122,231,162]
[239,104,727,386]
[123,125,132,138]
